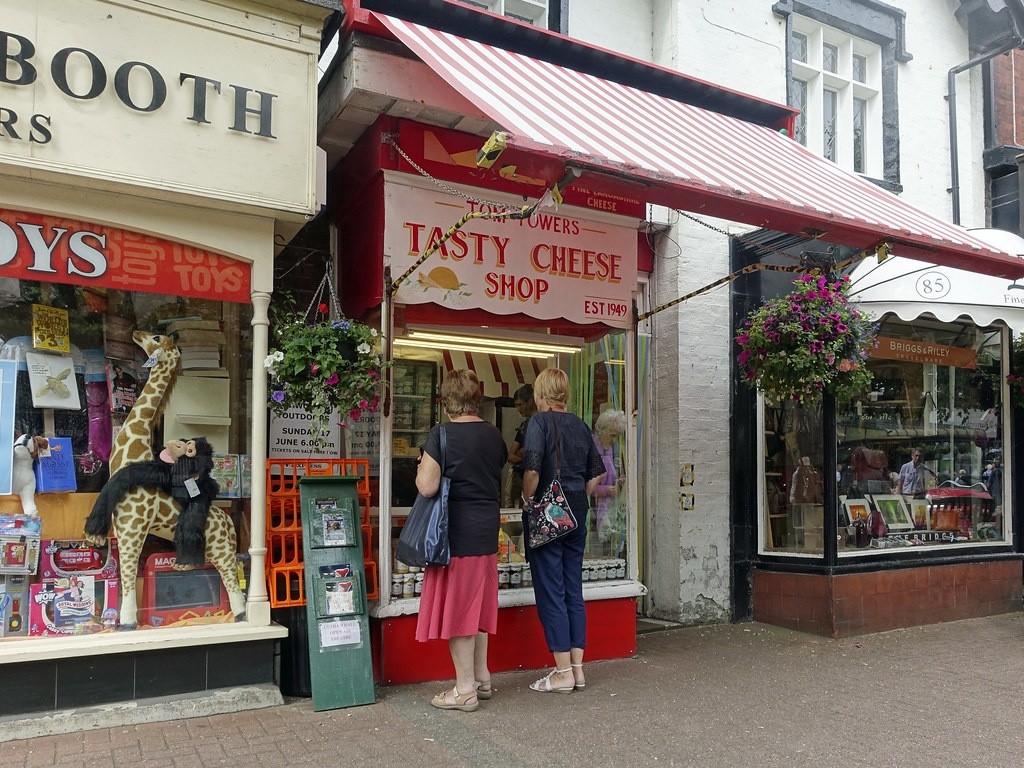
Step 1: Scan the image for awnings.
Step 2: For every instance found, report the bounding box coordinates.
[369,8,1024,280]
[844,227,1024,333]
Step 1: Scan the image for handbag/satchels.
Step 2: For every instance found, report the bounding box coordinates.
[394,423,451,568]
[527,479,578,549]
[851,447,888,487]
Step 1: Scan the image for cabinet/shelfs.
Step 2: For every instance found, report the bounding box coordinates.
[392,357,438,460]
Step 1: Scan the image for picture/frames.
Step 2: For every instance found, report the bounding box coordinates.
[869,492,916,530]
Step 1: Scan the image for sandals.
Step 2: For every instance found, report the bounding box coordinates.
[431,680,492,712]
[528,664,586,695]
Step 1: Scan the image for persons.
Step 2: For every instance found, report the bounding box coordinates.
[954,462,1002,506]
[887,447,927,500]
[522,368,607,693]
[590,409,627,555]
[508,385,538,508]
[113,364,140,413]
[416,368,508,711]
[790,457,818,504]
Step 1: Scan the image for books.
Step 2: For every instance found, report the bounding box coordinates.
[31,304,70,355]
[32,437,77,494]
[103,314,141,415]
[26,352,81,410]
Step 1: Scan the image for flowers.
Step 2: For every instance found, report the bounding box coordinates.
[262,303,382,453]
[731,268,882,407]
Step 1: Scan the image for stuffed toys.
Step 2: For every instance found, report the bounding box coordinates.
[82,331,247,631]
[12,430,48,516]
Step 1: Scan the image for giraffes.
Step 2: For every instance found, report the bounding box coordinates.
[89,330,278,631]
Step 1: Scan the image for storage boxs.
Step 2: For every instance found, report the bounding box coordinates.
[0,574,30,637]
[38,538,119,583]
[0,514,43,576]
[34,437,78,494]
[28,578,120,637]
